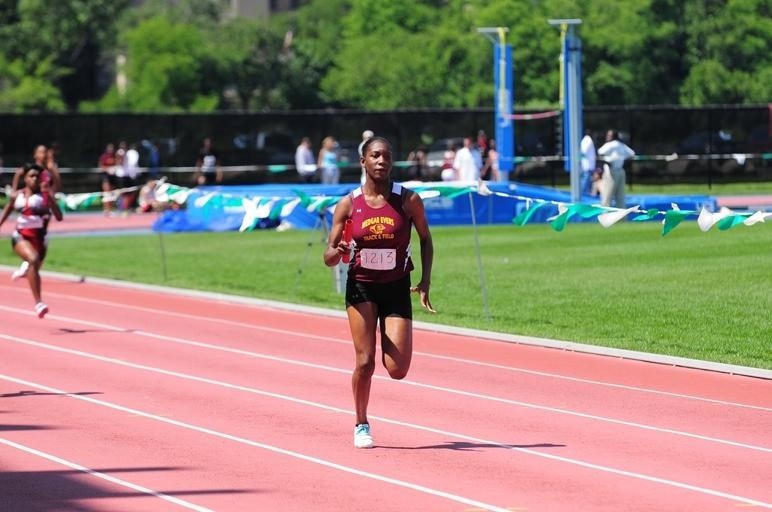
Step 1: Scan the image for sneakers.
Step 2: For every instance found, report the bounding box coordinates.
[10,261,31,280]
[33,302,50,320]
[352,420,377,451]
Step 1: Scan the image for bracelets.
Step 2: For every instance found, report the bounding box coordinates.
[422,279,430,285]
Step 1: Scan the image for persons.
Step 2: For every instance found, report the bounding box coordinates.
[96,137,164,213]
[294,137,318,183]
[581,128,636,209]
[324,136,436,449]
[358,130,375,185]
[318,136,341,184]
[0,162,54,319]
[196,137,223,185]
[407,129,498,183]
[12,143,62,194]
